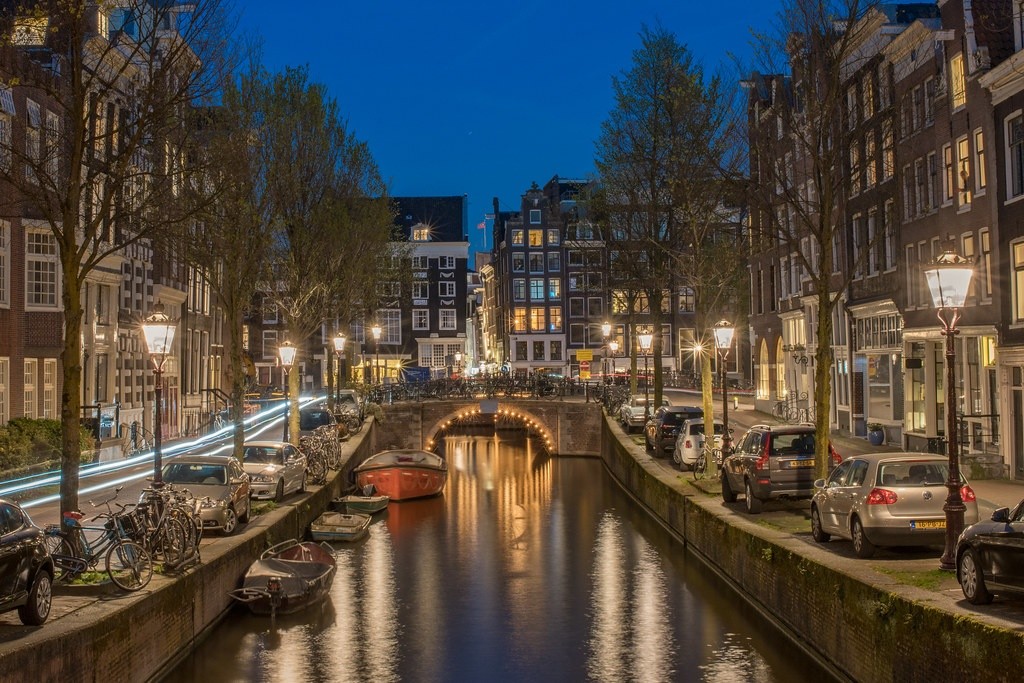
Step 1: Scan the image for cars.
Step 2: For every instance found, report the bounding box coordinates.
[673,418,737,471]
[337,388,364,418]
[952,496,1024,605]
[0,498,55,627]
[282,406,341,449]
[811,450,979,559]
[142,455,252,534]
[619,395,672,432]
[242,440,308,502]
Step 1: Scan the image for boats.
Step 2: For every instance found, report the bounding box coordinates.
[309,509,373,543]
[351,449,450,502]
[330,484,393,514]
[227,538,339,619]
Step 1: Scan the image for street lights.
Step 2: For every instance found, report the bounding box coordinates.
[371,324,382,384]
[332,333,347,413]
[601,320,611,384]
[139,297,180,491]
[277,340,299,442]
[921,247,976,573]
[638,331,654,434]
[609,342,619,375]
[713,318,736,468]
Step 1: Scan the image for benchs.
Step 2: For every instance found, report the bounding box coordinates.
[322,522,356,527]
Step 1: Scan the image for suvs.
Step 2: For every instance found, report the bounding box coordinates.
[645,404,703,454]
[719,422,842,514]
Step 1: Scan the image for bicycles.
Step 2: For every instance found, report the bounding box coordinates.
[691,437,739,482]
[39,483,211,593]
[297,420,344,483]
[364,370,635,415]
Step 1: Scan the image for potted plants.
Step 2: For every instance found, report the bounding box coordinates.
[867,422,885,446]
